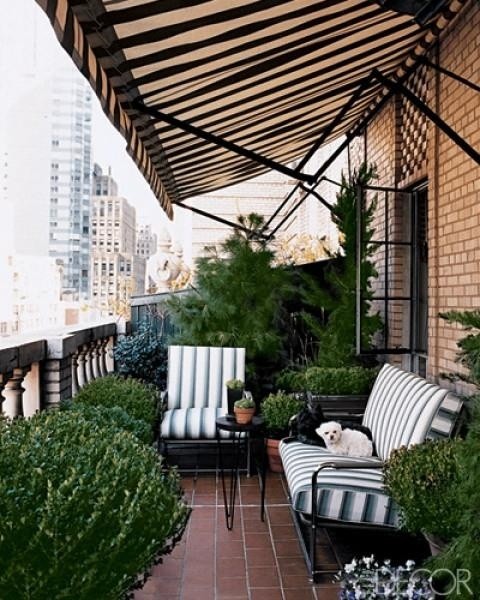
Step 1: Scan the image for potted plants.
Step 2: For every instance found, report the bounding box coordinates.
[226,378,306,474]
[379,434,480,562]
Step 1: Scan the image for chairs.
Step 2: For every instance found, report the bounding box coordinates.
[156,343,252,479]
[278,364,469,587]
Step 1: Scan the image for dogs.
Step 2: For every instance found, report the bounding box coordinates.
[282,404,378,458]
[314,420,374,459]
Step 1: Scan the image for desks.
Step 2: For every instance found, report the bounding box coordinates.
[216,416,265,530]
